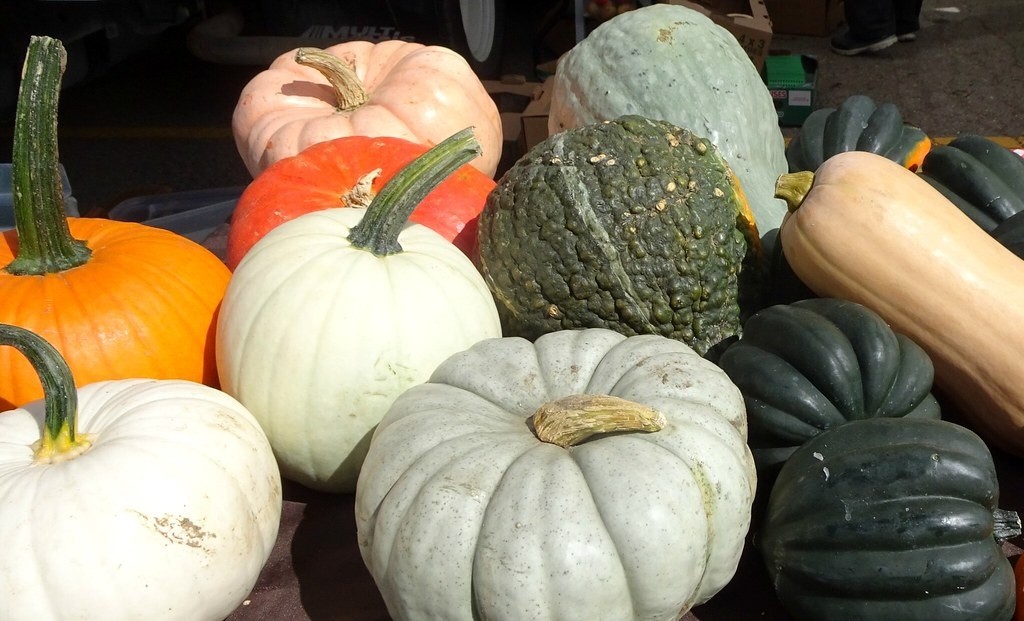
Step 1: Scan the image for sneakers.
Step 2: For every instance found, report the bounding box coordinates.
[831,30,917,56]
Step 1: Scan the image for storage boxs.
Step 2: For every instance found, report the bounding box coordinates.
[762,49,818,126]
[536,59,557,83]
[520,75,555,153]
[0,197,80,230]
[763,0,844,37]
[665,0,773,76]
[0,163,71,200]
[479,80,539,180]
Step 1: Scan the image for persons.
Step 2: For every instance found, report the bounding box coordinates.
[830,0,922,56]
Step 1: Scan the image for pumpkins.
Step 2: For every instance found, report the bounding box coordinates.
[0,4,1024,621]
